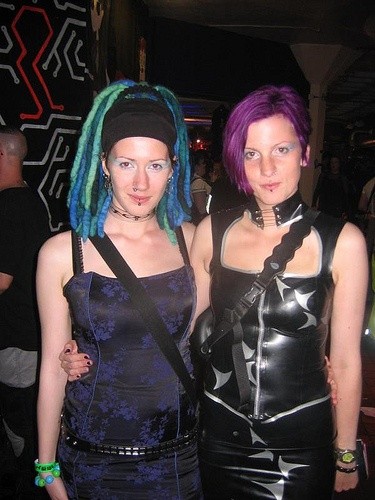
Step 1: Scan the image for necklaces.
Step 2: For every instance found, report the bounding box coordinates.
[108,200,156,223]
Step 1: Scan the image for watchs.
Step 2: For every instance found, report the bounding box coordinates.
[336,448,358,463]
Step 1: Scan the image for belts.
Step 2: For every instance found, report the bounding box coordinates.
[58,412,200,459]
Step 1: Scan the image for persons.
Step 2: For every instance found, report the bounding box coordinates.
[0,79,375,500]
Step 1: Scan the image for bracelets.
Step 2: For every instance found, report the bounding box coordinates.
[336,465,359,473]
[34,458,57,472]
[34,469,62,487]
